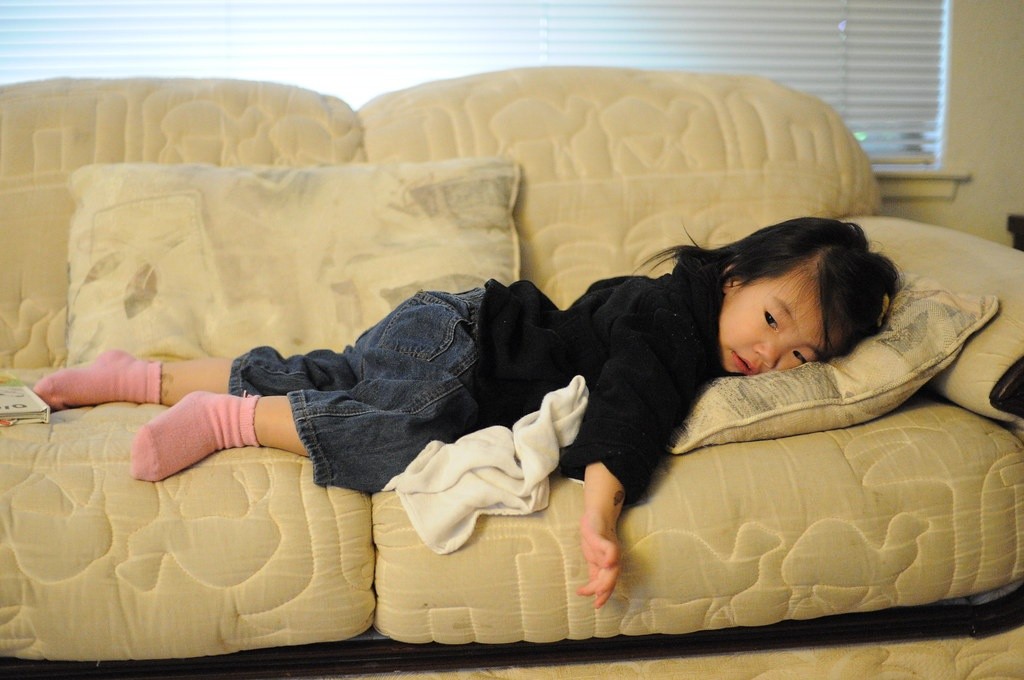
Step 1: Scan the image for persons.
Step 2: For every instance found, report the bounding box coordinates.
[35,217,906,608]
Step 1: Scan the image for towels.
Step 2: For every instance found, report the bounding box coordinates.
[380,375,590,555]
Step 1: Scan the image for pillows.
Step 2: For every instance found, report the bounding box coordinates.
[65,159,520,366]
[666,272,998,454]
[0,66,1024,660]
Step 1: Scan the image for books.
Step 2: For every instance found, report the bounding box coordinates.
[0,373,50,427]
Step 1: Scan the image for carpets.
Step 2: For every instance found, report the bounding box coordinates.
[243,627,1024,680]
